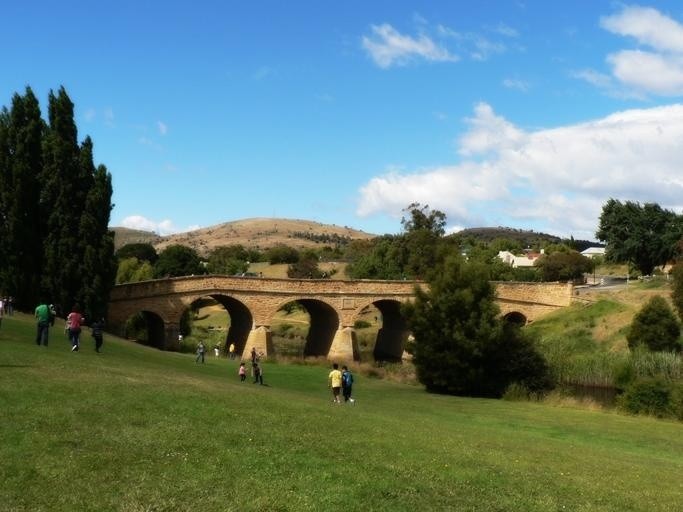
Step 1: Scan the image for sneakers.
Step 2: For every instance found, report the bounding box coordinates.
[334,399,356,406]
[72,345,78,351]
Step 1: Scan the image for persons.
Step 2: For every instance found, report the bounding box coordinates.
[327,362,357,405]
[309,272,327,279]
[403,275,407,281]
[239,347,268,386]
[0,296,14,316]
[28,302,104,353]
[192,338,235,366]
[240,271,263,278]
[179,332,183,342]
